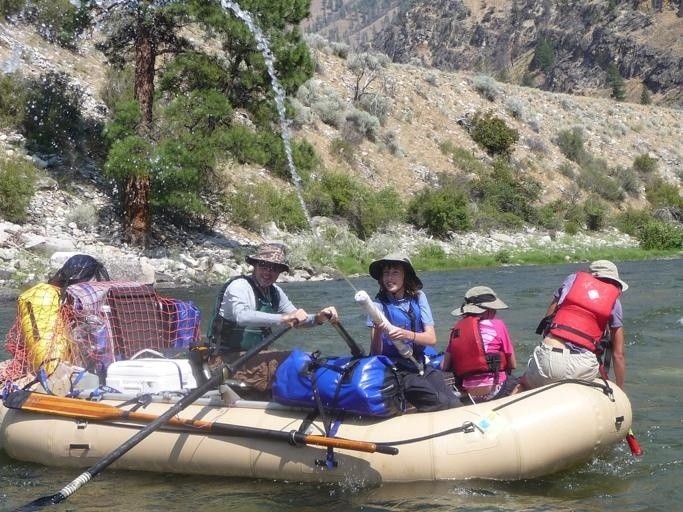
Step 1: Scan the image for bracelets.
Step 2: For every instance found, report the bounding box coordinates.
[412,330,416,344]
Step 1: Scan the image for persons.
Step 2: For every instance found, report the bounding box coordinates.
[366,253,464,412]
[511,260,629,395]
[440,286,524,402]
[208,242,338,392]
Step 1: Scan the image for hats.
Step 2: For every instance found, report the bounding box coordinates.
[369,252,425,290]
[245,241,290,272]
[588,259,630,293]
[451,285,511,316]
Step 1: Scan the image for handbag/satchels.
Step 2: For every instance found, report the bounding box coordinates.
[273,345,408,418]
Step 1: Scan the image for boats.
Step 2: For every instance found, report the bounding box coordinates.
[0,358,632,485]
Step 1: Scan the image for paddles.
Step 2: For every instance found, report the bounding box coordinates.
[2,391,377,454]
